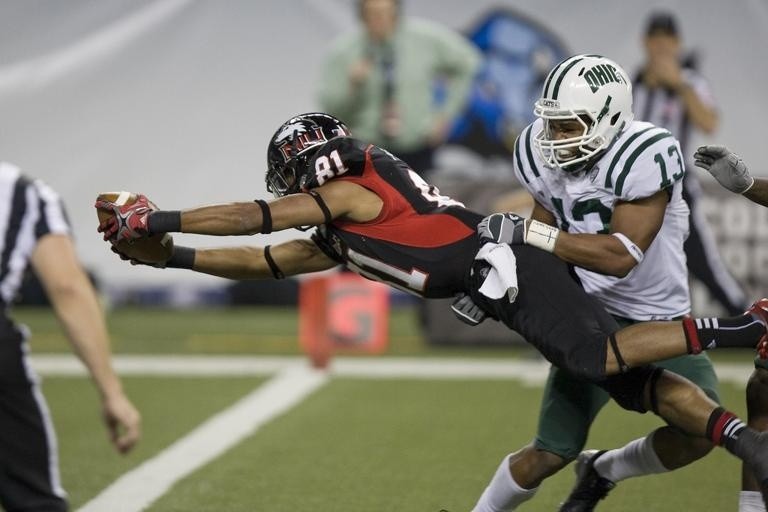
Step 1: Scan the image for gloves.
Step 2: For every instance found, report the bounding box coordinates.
[93,191,173,271]
[477,211,527,248]
[448,293,486,326]
[694,144,754,195]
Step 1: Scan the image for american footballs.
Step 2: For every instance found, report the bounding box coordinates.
[96,192,175,268]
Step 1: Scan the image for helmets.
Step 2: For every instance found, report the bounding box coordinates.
[265,112,354,231]
[533,53,635,171]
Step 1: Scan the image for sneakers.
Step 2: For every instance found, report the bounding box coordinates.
[744,297,768,369]
[559,448,615,512]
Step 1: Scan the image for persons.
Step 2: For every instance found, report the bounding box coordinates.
[452,52,720,512]
[693,144,768,207]
[737,330,768,511]
[96,113,767,501]
[314,1,480,184]
[629,12,752,318]
[0,156,141,511]
